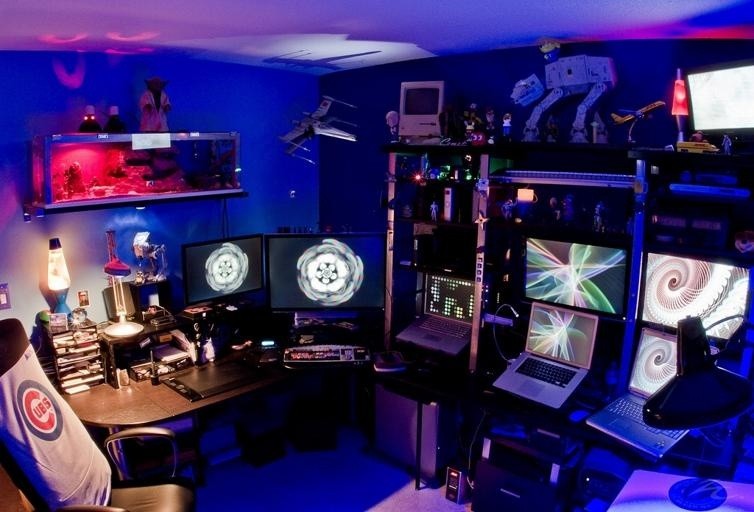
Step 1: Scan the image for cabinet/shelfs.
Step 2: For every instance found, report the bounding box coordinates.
[52,325,106,396]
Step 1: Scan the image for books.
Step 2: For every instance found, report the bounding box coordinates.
[155,343,189,365]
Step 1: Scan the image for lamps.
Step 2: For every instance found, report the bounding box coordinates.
[671,68,688,143]
[103,229,144,338]
[47,237,72,322]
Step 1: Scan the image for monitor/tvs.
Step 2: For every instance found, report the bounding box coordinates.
[179,234,263,303]
[265,234,386,311]
[524,233,632,319]
[638,241,752,361]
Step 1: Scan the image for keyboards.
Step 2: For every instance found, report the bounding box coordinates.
[284,346,371,368]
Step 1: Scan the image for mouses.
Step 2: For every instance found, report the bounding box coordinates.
[381,350,394,369]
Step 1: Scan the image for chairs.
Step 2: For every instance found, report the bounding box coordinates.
[0,318,197,512]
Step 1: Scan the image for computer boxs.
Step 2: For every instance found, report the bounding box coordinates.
[473,418,591,511]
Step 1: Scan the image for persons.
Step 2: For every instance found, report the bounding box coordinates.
[721,134,732,155]
[502,113,512,141]
[430,201,438,224]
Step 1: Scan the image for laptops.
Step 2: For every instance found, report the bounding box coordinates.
[587,319,720,461]
[397,272,475,355]
[492,302,600,409]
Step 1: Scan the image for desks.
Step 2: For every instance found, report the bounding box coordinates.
[56,363,270,481]
